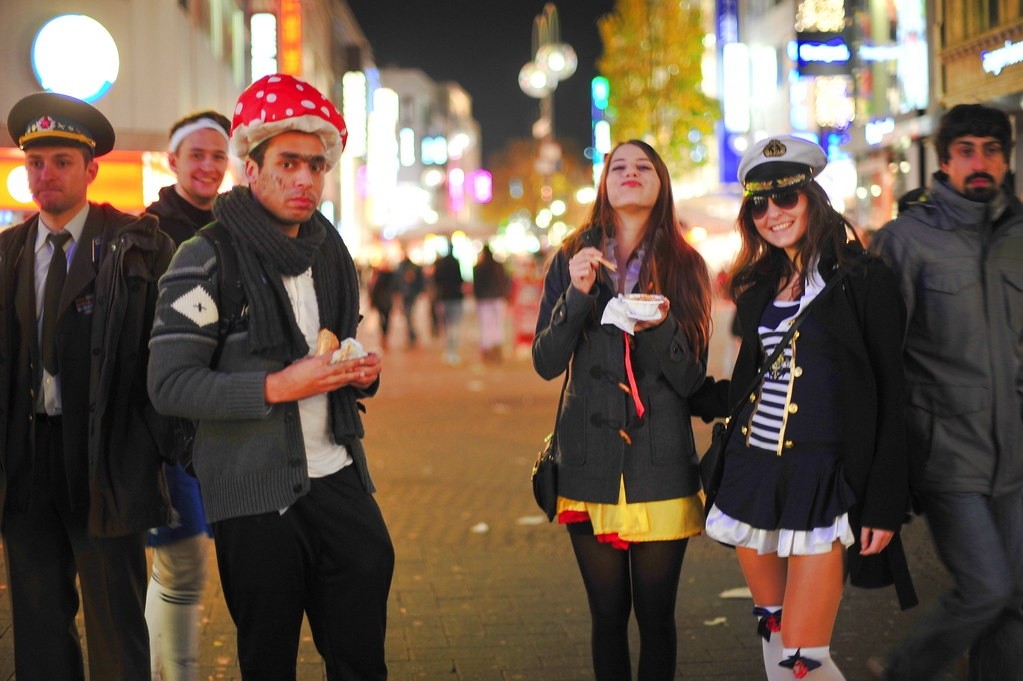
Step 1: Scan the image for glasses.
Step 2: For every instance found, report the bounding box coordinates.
[747,190,803,218]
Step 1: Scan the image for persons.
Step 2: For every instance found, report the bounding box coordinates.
[871,100,1022,680]
[139,112,235,680]
[532,139,714,681]
[0,94,180,681]
[146,72,396,681]
[368,238,513,370]
[705,137,916,681]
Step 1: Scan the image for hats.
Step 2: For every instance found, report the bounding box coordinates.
[230,75,348,172]
[736,136,829,193]
[7,92,116,157]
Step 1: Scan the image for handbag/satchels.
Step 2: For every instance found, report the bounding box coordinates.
[533,434,562,524]
[700,422,725,495]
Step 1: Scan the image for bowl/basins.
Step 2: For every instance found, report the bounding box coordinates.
[626,293,664,317]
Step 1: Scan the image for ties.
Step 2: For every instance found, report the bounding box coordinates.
[41,231,70,377]
